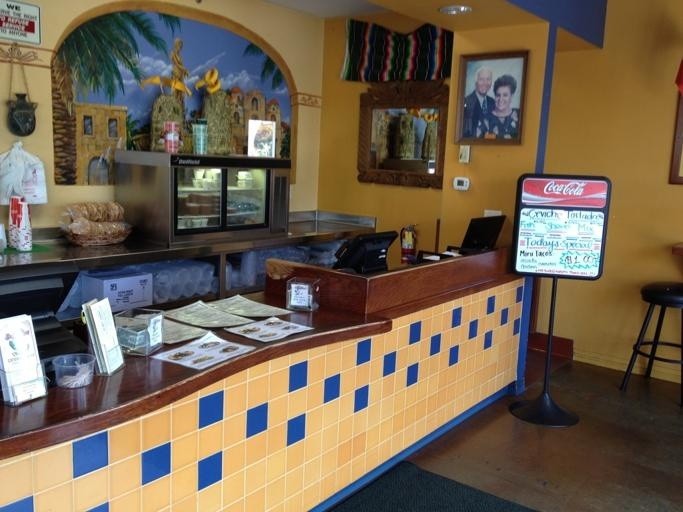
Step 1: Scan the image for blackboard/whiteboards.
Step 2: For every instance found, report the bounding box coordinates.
[514,206,606,280]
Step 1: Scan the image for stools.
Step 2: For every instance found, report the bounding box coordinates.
[618,280,682,407]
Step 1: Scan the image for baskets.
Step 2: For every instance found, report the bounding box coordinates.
[65,229,131,246]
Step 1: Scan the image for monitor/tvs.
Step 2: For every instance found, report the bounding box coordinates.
[460,215,507,255]
[332,231,399,275]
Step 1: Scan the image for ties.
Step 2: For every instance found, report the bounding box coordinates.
[482,100,487,113]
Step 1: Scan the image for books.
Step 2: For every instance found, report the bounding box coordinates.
[0,311,43,406]
[82,294,119,376]
[147,293,313,372]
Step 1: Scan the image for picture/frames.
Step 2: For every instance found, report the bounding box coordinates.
[356,49,527,190]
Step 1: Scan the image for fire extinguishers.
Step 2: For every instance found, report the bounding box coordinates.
[400,224,419,265]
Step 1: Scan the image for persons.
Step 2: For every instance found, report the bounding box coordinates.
[472,75,521,139]
[464,67,495,137]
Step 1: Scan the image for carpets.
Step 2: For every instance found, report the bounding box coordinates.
[319,459,541,511]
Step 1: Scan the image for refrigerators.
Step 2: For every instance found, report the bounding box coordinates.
[112,150,292,249]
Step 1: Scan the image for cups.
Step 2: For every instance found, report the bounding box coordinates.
[8,196,32,252]
[191,117,209,155]
[162,122,180,153]
